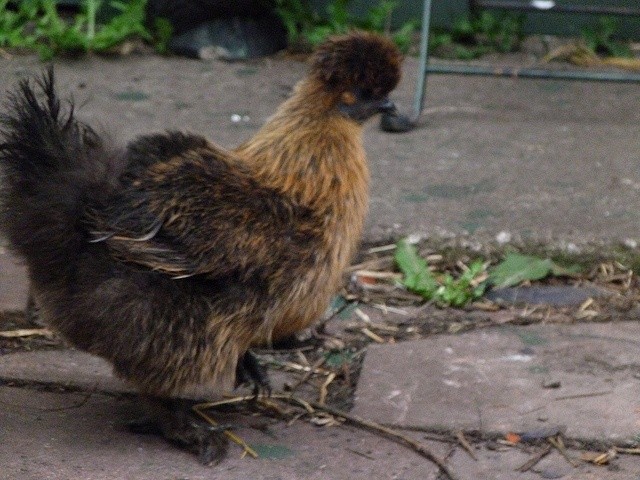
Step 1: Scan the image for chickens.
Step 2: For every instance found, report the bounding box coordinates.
[0,27,404,465]
